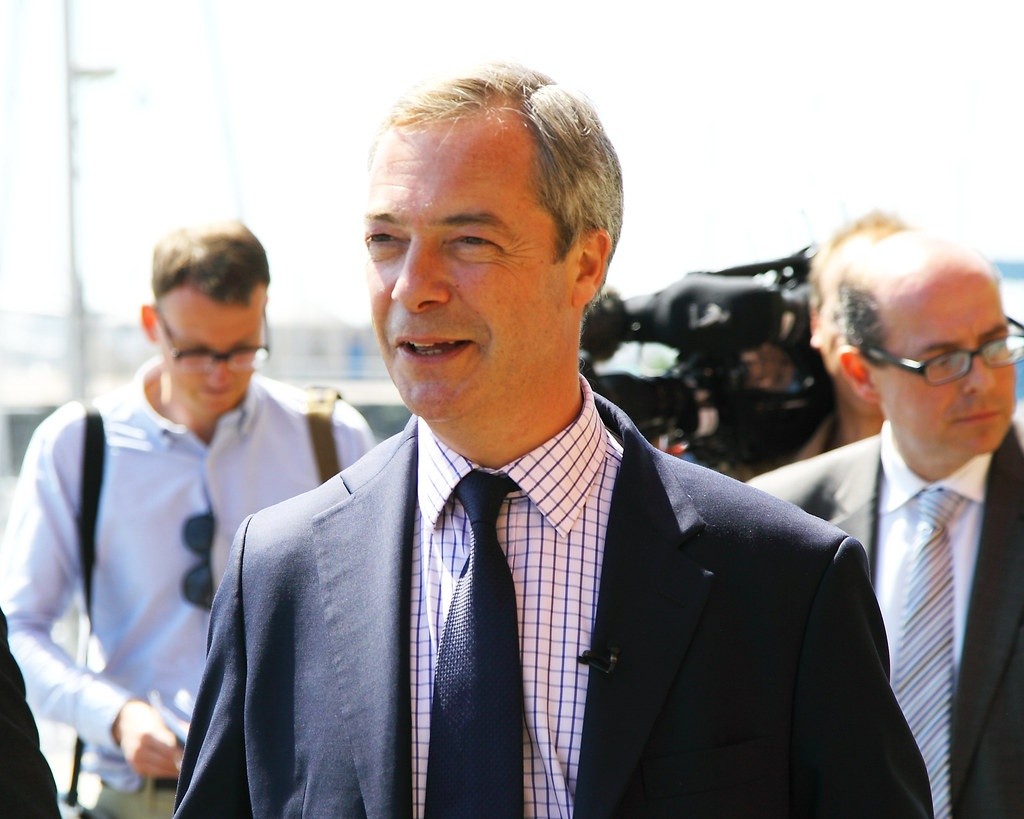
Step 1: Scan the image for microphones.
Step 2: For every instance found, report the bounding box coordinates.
[583,649,618,667]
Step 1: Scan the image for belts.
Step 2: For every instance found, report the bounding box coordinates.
[142,776,178,793]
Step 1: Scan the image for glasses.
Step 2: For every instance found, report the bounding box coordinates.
[155,304,272,374]
[860,314,1024,387]
[182,509,215,611]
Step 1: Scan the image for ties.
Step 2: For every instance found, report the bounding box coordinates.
[424,469,524,819]
[891,487,965,819]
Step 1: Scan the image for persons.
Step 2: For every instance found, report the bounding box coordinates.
[173,59,933,819]
[0,218,382,819]
[743,211,1024,819]
